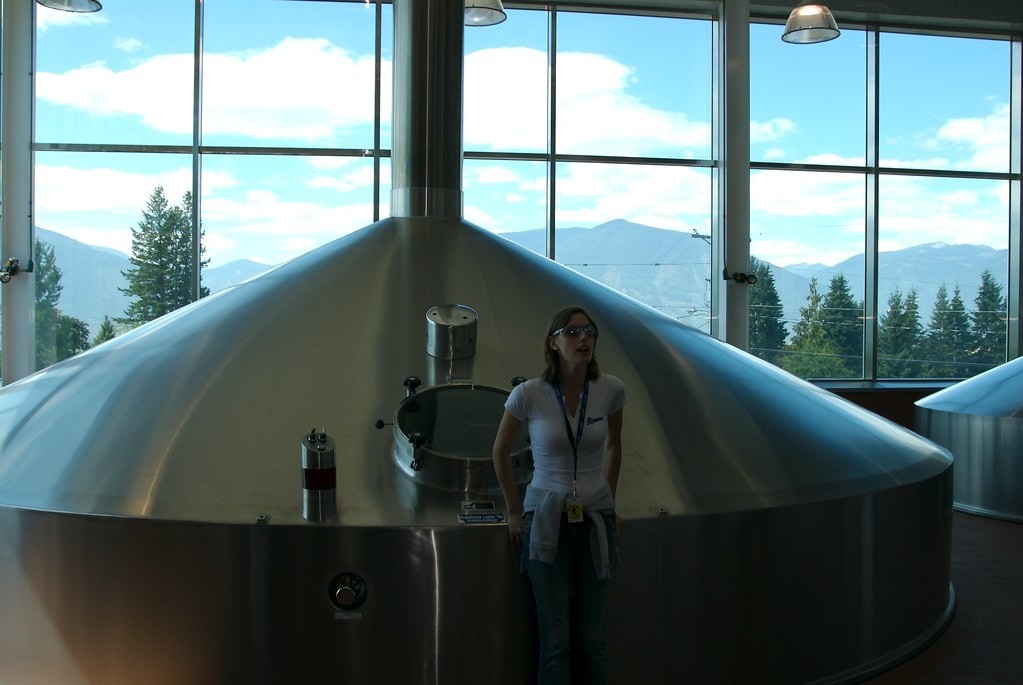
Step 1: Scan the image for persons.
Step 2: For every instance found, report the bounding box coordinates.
[492,306,626,685]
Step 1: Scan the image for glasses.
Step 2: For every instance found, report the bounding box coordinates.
[552,325,597,339]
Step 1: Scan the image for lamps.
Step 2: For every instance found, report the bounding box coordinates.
[35,0,102,14]
[464,0,507,26]
[781,0,842,45]
